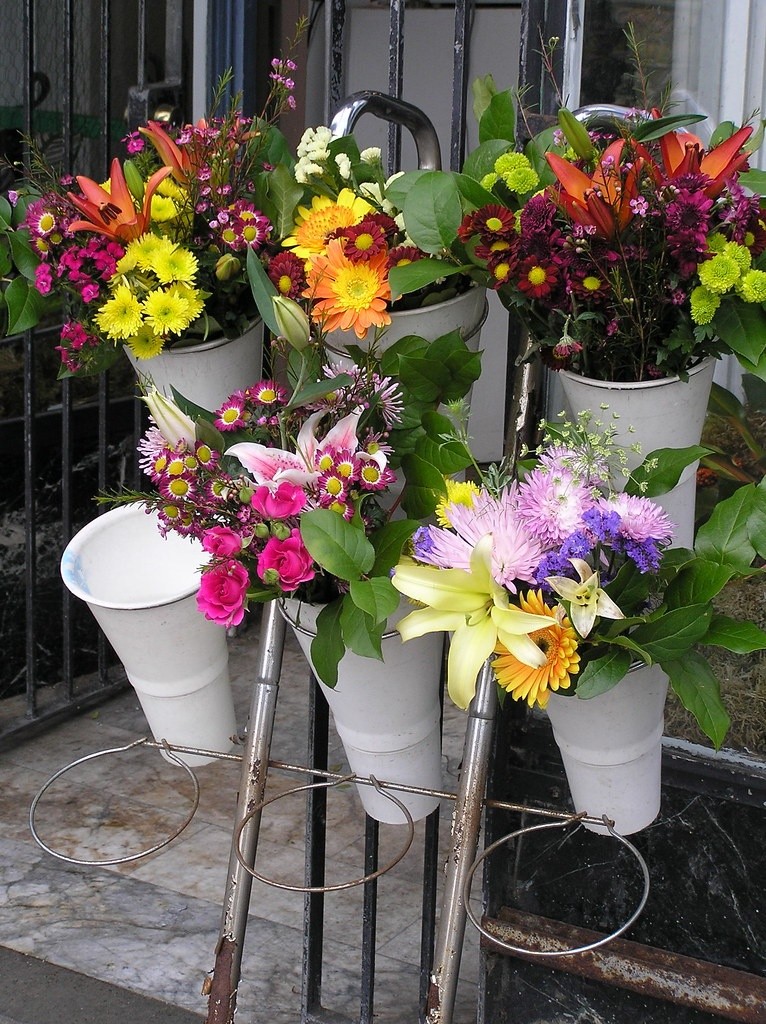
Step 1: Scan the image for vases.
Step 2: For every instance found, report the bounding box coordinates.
[318,284,485,485]
[546,660,669,836]
[61,497,238,767]
[559,354,716,551]
[122,314,264,413]
[279,596,444,824]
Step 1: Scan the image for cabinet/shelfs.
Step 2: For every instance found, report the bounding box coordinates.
[479,681,766,1024]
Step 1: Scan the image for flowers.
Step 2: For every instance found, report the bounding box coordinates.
[0,15,766,753]
